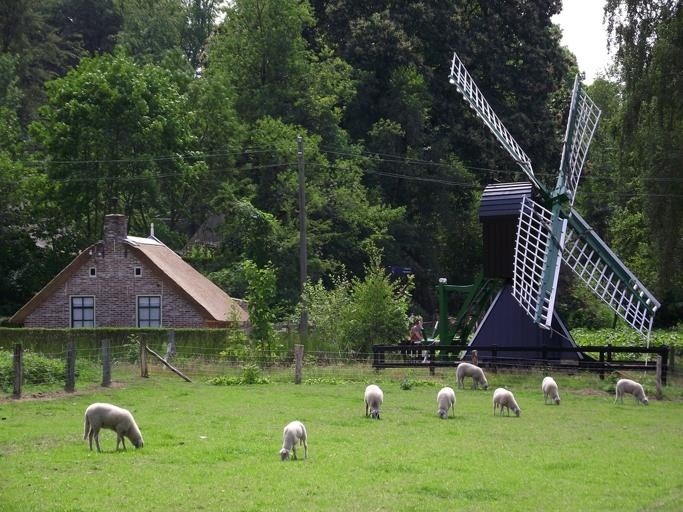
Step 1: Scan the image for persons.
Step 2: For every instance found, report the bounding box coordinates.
[410,320,429,362]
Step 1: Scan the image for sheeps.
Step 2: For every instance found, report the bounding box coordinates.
[82,402,145,453]
[491,387,523,418]
[541,376,561,406]
[278,420,309,461]
[363,384,384,421]
[613,378,650,407]
[436,386,457,420]
[454,362,490,392]
